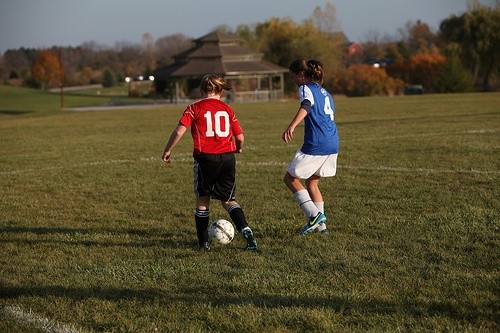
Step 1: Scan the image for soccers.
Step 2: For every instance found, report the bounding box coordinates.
[208,218,235,246]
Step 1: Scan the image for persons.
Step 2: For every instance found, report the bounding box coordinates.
[162,74,258,252]
[282,59,339,237]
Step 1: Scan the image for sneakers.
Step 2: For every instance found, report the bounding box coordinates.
[301,211,327,235]
[317,223,326,233]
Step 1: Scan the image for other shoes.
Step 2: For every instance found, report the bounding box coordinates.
[242,226,257,249]
[200,241,210,250]
